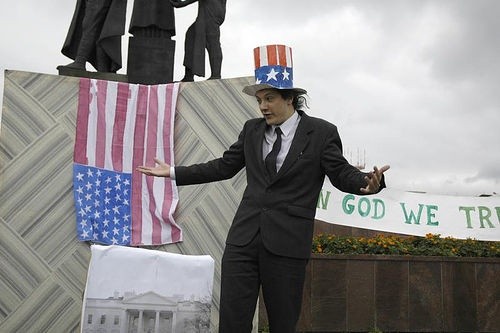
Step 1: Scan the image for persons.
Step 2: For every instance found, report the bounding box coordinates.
[170,1,227,82]
[58,1,128,74]
[129,0,177,39]
[135,45,390,333]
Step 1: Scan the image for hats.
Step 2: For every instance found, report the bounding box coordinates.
[242,45,307,96]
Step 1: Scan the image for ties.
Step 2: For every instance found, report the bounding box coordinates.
[264,127,282,178]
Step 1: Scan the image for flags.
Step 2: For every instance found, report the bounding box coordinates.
[72,77,184,246]
